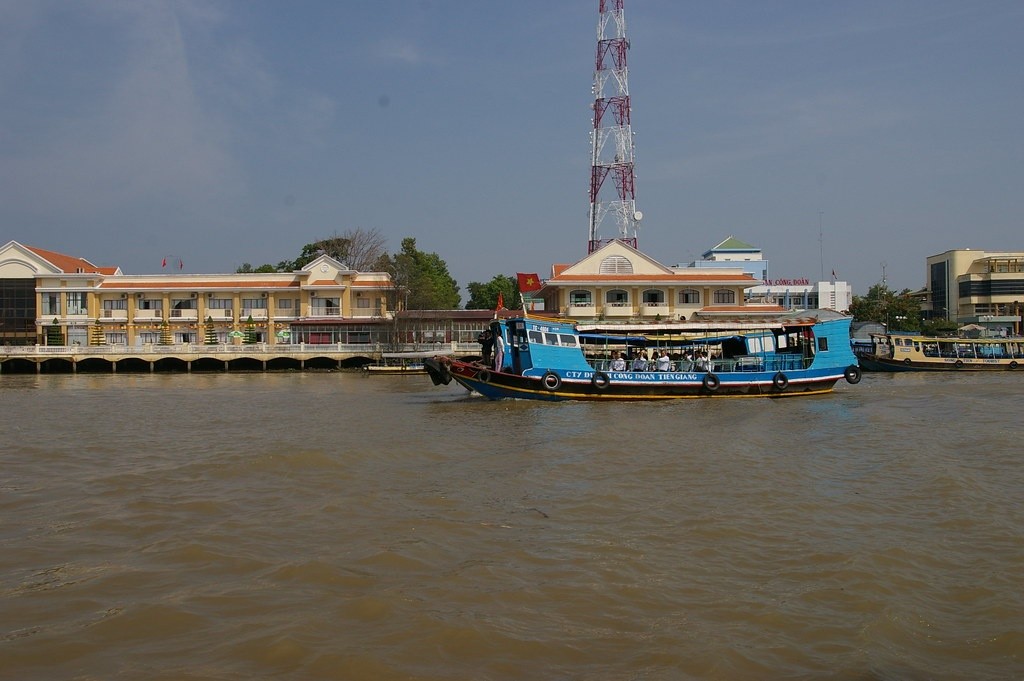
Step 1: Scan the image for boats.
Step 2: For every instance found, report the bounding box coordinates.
[849,331,1024,373]
[420,272,865,398]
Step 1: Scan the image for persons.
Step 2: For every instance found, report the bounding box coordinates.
[479,330,493,368]
[494,331,505,373]
[607,349,714,372]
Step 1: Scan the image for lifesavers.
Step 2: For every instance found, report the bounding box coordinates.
[591,371,610,390]
[955,360,963,368]
[773,372,789,391]
[541,371,562,392]
[903,358,911,365]
[844,365,861,384]
[423,358,452,386]
[702,373,720,391]
[1009,361,1018,369]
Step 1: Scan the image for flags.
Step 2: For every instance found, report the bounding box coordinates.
[495,292,503,312]
[832,269,838,279]
[180,259,184,269]
[162,259,166,267]
[516,272,541,293]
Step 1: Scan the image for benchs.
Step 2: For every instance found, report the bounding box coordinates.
[735,357,764,372]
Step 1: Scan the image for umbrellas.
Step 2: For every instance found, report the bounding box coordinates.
[276,330,290,342]
[958,324,987,331]
[228,331,245,338]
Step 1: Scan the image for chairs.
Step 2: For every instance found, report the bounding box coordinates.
[667,362,671,372]
[623,362,626,372]
[689,363,694,372]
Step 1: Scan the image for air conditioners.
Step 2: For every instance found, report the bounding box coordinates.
[260,293,267,298]
[207,293,215,299]
[119,293,128,299]
[136,293,145,299]
[309,291,317,298]
[190,292,197,299]
[355,291,362,298]
